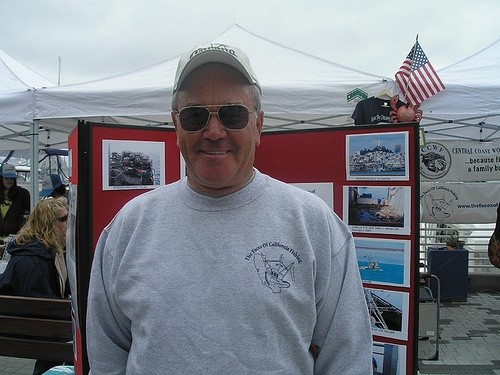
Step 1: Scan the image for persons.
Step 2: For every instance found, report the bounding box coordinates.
[87,42,372,375]
[40,174,70,202]
[0,163,30,260]
[0,196,71,299]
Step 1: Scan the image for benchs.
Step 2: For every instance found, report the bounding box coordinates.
[0,295,75,362]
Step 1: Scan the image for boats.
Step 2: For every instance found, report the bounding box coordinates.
[368,261,378,270]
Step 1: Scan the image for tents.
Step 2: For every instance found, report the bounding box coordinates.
[38,148,69,175]
[0,22,396,213]
[0,47,57,96]
[396,39,500,142]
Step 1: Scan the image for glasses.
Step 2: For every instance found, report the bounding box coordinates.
[55,213,69,222]
[174,104,258,132]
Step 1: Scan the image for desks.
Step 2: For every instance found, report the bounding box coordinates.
[426,246,469,303]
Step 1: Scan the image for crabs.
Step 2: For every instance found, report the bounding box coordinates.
[389,95,423,123]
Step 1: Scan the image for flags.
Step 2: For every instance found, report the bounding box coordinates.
[394,36,445,107]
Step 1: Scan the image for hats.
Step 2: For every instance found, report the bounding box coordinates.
[172,41,264,97]
[1,163,18,178]
